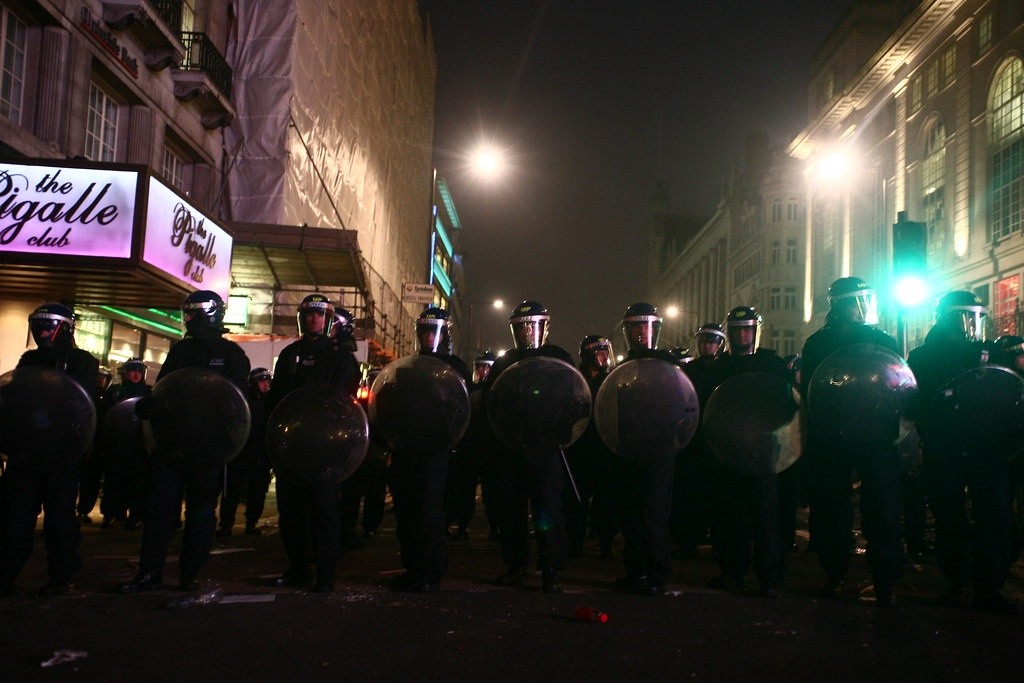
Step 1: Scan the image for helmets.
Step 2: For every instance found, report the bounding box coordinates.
[472,351,496,382]
[620,303,663,352]
[297,294,332,338]
[95,365,114,393]
[784,354,803,389]
[669,347,694,368]
[695,324,727,361]
[994,334,1024,374]
[250,367,272,394]
[579,334,616,373]
[724,307,762,355]
[181,290,225,336]
[330,307,355,338]
[828,275,879,325]
[122,359,147,389]
[415,308,453,354]
[508,301,550,351]
[26,303,75,354]
[934,288,990,341]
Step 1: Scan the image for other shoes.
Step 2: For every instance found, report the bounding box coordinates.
[1,515,1023,618]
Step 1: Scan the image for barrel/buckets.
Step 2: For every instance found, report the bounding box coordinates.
[542,567,560,592]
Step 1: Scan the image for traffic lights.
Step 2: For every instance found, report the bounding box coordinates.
[891,221,929,316]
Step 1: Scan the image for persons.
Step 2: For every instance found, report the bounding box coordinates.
[482,301,575,591]
[388,308,496,593]
[612,302,679,593]
[673,324,725,559]
[0,303,101,597]
[699,307,794,597]
[217,367,270,537]
[265,293,388,594]
[906,291,1024,610]
[799,277,905,609]
[119,290,249,594]
[77,358,153,530]
[567,335,619,561]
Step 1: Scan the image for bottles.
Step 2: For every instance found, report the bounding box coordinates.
[562,600,609,624]
